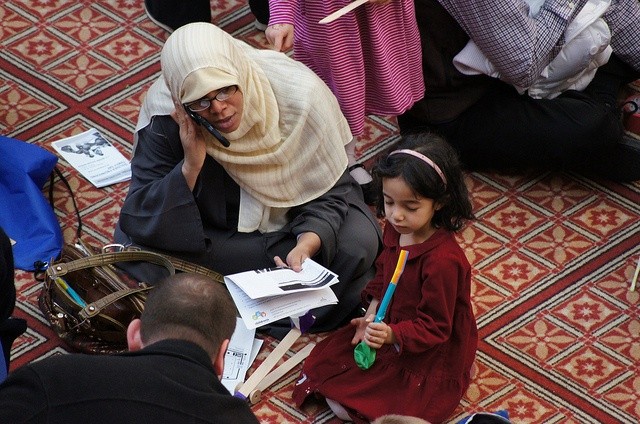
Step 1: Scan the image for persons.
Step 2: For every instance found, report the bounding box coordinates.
[397,1,638,183]
[0,273,258,424]
[145,1,269,36]
[266,0,425,205]
[291,136,478,424]
[113,21,385,340]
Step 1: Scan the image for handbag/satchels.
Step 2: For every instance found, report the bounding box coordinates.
[541,91,638,151]
[0,134,81,273]
[37,243,176,357]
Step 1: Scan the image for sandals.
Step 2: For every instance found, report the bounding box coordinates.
[348,163,379,206]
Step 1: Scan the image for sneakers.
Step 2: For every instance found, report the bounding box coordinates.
[255,18,268,31]
[144,0,213,35]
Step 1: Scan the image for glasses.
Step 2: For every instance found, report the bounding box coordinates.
[182,85,239,112]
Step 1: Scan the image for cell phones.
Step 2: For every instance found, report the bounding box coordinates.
[182,102,230,148]
